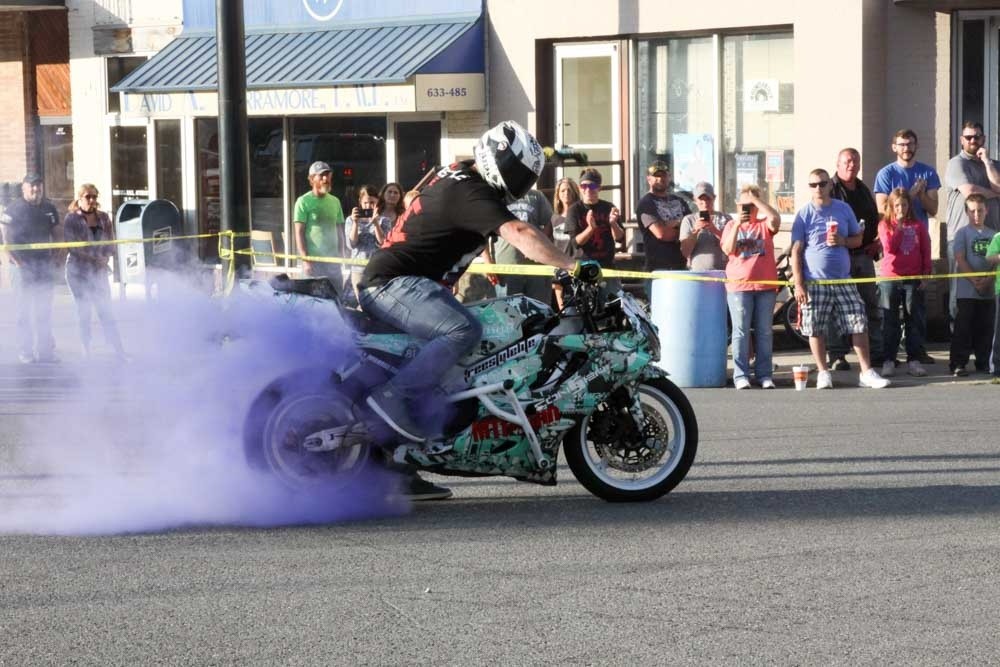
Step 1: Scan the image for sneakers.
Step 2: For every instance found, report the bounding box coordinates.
[908,360,927,375]
[881,361,895,376]
[367,382,426,443]
[384,473,452,500]
[858,368,891,388]
[816,371,833,389]
[735,378,751,389]
[761,380,776,389]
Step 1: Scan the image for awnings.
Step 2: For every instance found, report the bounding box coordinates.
[110,14,485,117]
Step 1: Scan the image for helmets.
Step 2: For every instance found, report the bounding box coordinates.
[474,120,545,205]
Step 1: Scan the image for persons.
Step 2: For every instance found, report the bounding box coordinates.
[353,117,604,502]
[547,177,581,261]
[634,157,692,301]
[677,180,734,272]
[378,183,406,229]
[342,182,392,305]
[789,168,892,390]
[479,189,555,307]
[872,128,941,365]
[944,120,1000,378]
[719,184,782,389]
[877,185,930,376]
[294,159,347,293]
[564,167,626,301]
[0,173,63,365]
[63,182,136,363]
[827,148,899,371]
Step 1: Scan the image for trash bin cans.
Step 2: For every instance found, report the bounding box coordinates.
[116,199,184,284]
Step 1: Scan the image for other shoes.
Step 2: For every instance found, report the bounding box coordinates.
[952,366,969,376]
[907,354,935,363]
[40,354,61,364]
[829,351,850,370]
[976,365,989,373]
[21,351,37,363]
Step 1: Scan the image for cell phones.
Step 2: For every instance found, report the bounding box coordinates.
[700,211,710,222]
[742,204,751,221]
[356,209,373,218]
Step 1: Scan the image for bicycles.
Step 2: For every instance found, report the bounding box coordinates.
[728,253,809,347]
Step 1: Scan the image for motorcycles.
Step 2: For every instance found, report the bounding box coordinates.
[239,250,702,504]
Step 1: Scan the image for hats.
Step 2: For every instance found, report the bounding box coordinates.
[309,162,332,175]
[694,182,715,199]
[23,173,42,187]
[648,160,669,176]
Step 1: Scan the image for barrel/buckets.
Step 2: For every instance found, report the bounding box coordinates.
[651,270,728,388]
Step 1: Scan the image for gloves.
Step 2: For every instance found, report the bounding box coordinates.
[570,258,602,284]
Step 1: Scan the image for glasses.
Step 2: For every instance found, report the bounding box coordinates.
[83,194,97,199]
[580,184,599,189]
[964,134,983,140]
[809,180,829,188]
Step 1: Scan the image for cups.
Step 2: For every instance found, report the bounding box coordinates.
[826,221,838,245]
[793,366,807,390]
